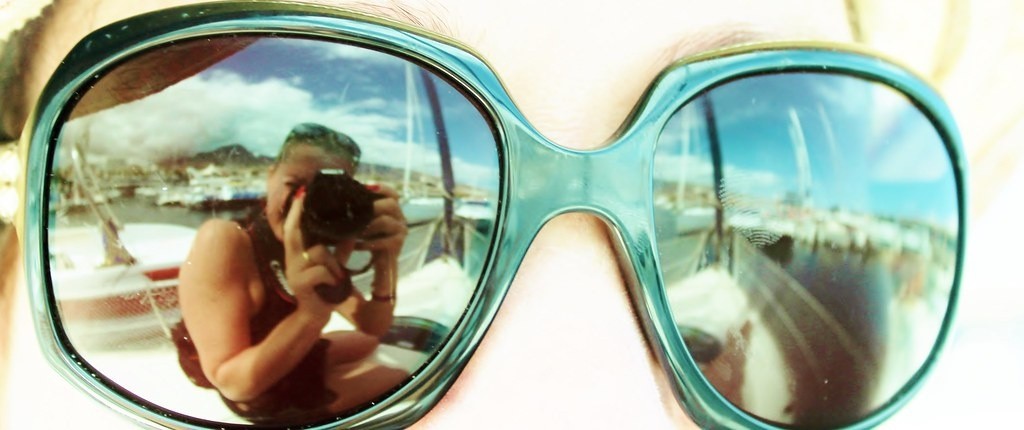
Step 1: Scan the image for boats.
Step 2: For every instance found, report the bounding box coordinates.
[674,189,939,274]
[51,146,271,212]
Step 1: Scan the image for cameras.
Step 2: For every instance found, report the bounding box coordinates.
[283,169,389,246]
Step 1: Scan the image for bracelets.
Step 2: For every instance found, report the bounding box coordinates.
[371,292,395,302]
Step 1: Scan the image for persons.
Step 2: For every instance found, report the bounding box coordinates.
[177,121,410,425]
[0,1,1024,430]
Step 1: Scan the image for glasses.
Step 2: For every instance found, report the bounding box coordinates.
[2,1,969,430]
[284,123,360,164]
